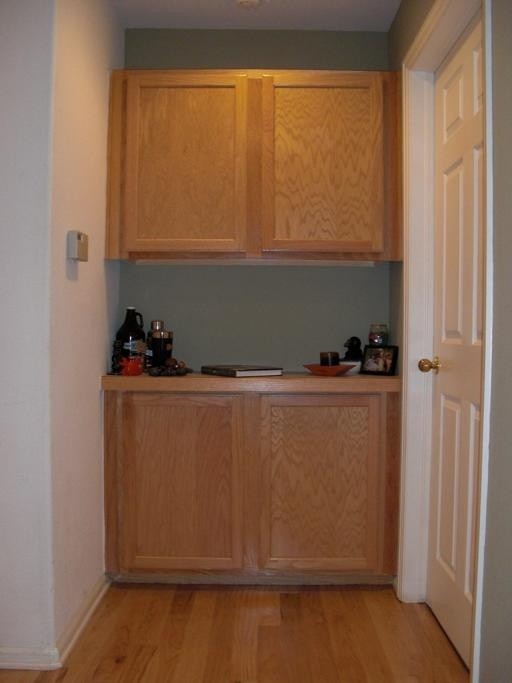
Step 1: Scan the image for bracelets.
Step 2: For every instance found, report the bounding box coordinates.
[340,362,361,375]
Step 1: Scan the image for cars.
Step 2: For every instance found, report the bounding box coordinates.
[319,351,340,365]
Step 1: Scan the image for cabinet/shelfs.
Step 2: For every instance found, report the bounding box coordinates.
[116,307,173,368]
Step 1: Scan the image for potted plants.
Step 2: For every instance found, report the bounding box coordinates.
[359,344,399,376]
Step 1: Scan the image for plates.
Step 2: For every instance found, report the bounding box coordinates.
[121,394,385,578]
[123,72,389,257]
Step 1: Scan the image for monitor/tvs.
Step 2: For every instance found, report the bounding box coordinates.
[201,362,283,377]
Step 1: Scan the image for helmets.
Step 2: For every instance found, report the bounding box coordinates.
[302,363,355,376]
